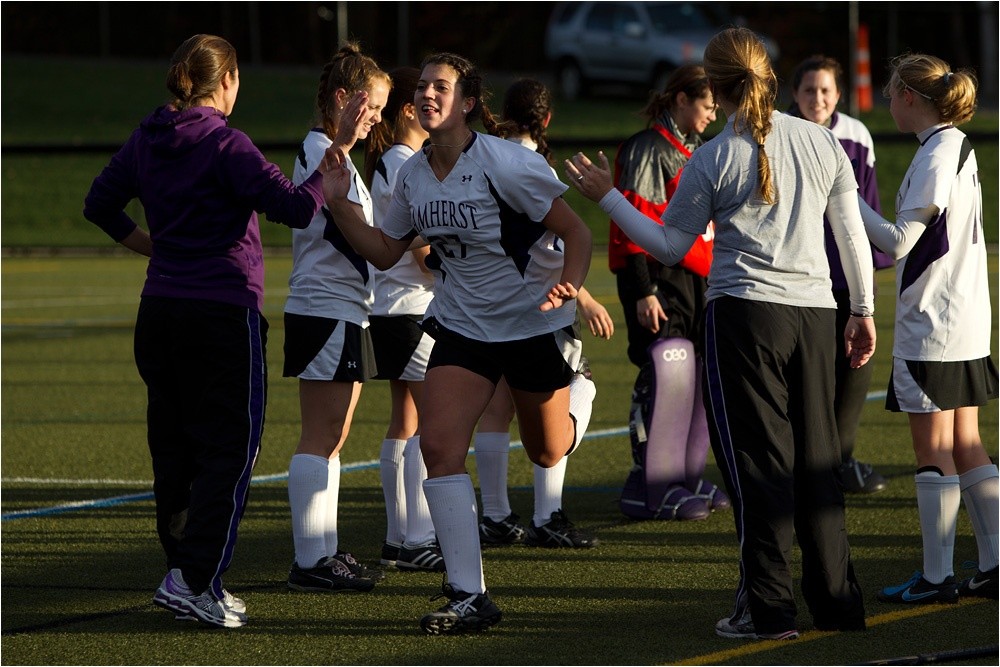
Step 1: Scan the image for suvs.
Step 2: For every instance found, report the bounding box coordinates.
[540,2,779,108]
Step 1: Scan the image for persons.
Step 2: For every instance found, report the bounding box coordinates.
[83,33,370,626]
[278,28,999,640]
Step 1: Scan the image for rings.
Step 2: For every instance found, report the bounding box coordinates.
[578,175,583,181]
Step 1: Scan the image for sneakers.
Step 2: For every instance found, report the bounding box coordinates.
[176,589,247,621]
[842,459,887,493]
[382,542,400,565]
[874,572,959,605]
[154,569,248,628]
[479,512,525,548]
[333,551,381,582]
[959,566,999,599]
[523,510,600,549]
[716,618,799,640]
[288,557,375,593]
[397,546,447,570]
[622,478,710,520]
[420,590,502,635]
[684,479,732,510]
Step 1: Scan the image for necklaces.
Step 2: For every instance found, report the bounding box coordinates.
[430,131,472,148]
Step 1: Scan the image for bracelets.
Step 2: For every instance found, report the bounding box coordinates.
[850,311,874,319]
